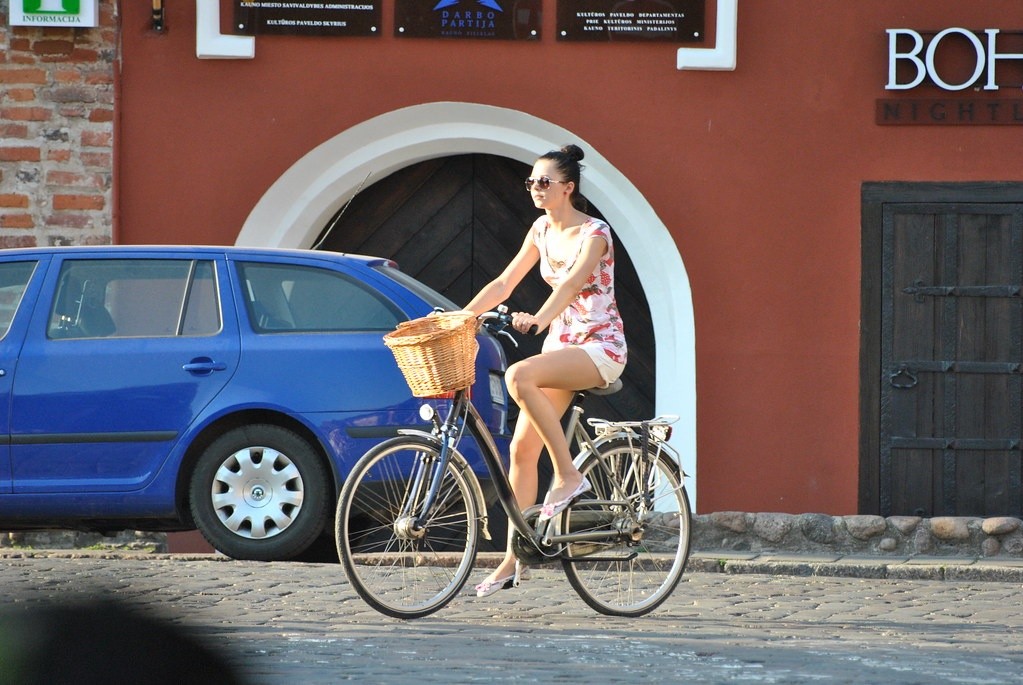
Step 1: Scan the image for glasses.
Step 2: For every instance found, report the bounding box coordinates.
[524,176,567,191]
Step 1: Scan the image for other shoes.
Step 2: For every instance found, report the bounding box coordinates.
[538,475,592,524]
[474,565,531,598]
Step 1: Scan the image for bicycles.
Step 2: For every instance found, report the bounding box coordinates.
[334,303,691,620]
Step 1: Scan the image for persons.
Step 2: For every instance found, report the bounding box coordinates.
[462,145,627,597]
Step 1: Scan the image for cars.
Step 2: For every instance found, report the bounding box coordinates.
[0,245,510,560]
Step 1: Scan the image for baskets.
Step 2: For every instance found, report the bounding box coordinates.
[382,310,478,398]
